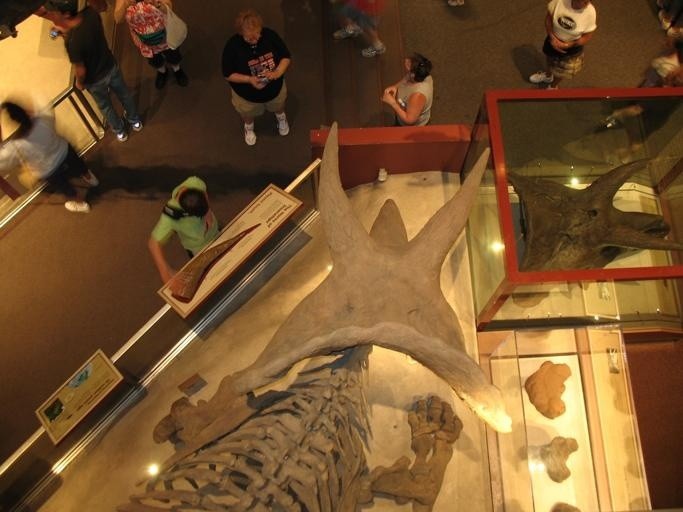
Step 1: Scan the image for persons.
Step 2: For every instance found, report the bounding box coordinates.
[0,100,98,213]
[48,0,143,141]
[448,0,464,7]
[529,0,597,89]
[659,0,683,39]
[333,1,387,58]
[604,38,682,127]
[148,176,220,285]
[382,54,434,127]
[222,10,292,145]
[114,1,187,90]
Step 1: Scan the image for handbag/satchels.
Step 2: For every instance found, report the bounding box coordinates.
[166,5,188,50]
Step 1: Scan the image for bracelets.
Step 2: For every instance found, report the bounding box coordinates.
[564,41,570,49]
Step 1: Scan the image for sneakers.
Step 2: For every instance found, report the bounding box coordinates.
[333,24,362,40]
[115,109,143,143]
[244,126,256,146]
[362,42,385,58]
[155,66,168,90]
[82,169,99,186]
[65,200,90,212]
[170,65,188,86]
[448,0,464,6]
[529,72,554,84]
[276,112,289,136]
[659,9,683,38]
